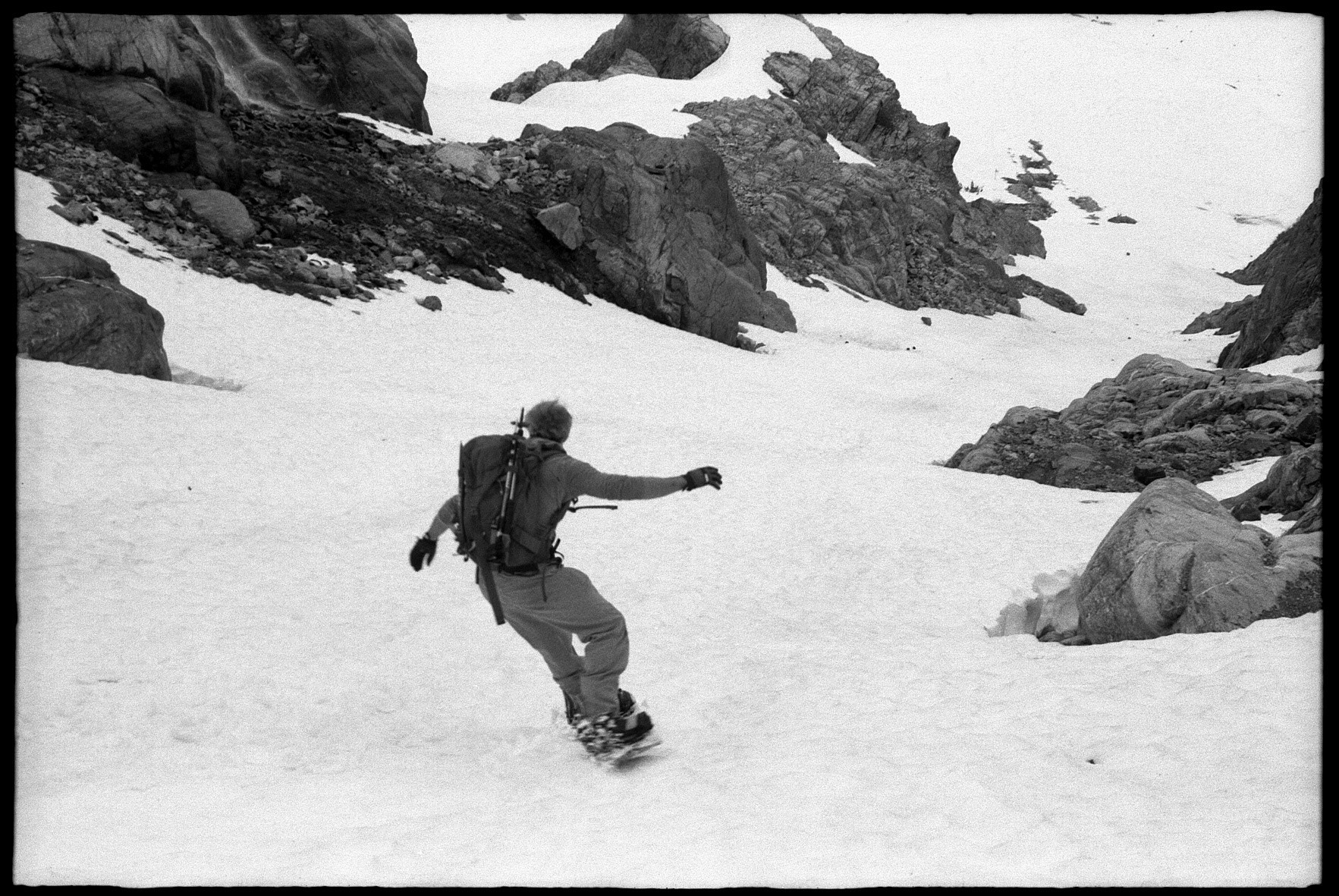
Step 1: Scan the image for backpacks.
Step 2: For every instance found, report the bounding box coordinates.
[456,433,565,566]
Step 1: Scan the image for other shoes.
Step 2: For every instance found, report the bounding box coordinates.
[617,686,636,710]
[593,711,654,745]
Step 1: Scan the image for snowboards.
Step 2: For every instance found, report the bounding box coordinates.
[566,685,664,768]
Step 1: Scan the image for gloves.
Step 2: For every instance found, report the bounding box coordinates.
[411,539,437,571]
[683,465,723,492]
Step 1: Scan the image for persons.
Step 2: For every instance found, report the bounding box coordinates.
[409,398,722,775]
